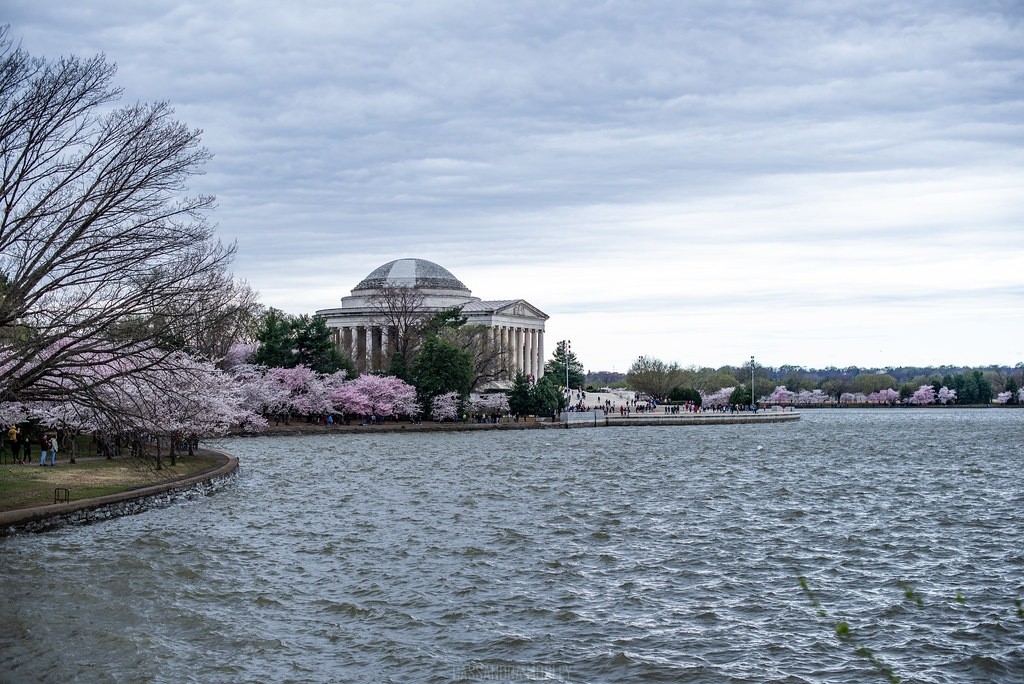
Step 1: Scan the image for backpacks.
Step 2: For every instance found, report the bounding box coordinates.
[47,439,54,450]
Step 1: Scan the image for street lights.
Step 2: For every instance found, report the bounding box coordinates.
[562,339,572,411]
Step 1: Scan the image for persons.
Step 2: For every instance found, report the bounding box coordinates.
[549,383,769,421]
[95,433,145,460]
[8,424,58,466]
[263,405,424,427]
[176,432,200,452]
[439,410,527,424]
